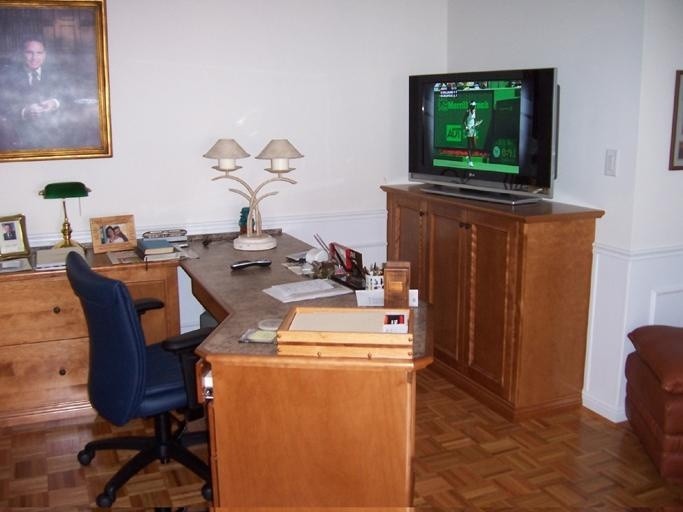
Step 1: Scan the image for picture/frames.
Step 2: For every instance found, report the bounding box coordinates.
[668,69,682,171]
[88,214,135,254]
[0,213,30,259]
[0,0,112,160]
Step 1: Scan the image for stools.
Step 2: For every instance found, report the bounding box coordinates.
[623,319,682,484]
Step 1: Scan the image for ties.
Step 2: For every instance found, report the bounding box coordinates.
[30,70,39,86]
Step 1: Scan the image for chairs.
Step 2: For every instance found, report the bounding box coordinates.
[65,248,217,512]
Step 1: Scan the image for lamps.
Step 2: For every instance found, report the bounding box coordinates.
[201,137,305,250]
[36,181,92,248]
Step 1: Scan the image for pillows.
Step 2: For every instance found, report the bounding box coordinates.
[627,324,681,393]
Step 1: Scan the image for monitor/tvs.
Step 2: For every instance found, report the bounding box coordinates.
[409,67,562,206]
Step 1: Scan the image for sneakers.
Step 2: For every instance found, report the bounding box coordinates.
[464,155,470,162]
[467,161,474,168]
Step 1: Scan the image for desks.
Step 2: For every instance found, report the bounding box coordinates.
[180,228,434,512]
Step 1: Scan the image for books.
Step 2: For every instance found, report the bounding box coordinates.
[262,278,353,303]
[0,258,32,273]
[33,246,86,271]
[138,229,190,262]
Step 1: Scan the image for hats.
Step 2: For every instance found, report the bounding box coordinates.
[469,100,477,107]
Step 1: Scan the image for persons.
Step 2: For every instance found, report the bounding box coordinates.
[2,224,16,240]
[105,225,128,243]
[463,99,483,167]
[0,34,102,151]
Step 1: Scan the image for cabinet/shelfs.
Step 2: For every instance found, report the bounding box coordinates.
[427,195,603,421]
[380,186,427,311]
[0,245,179,432]
